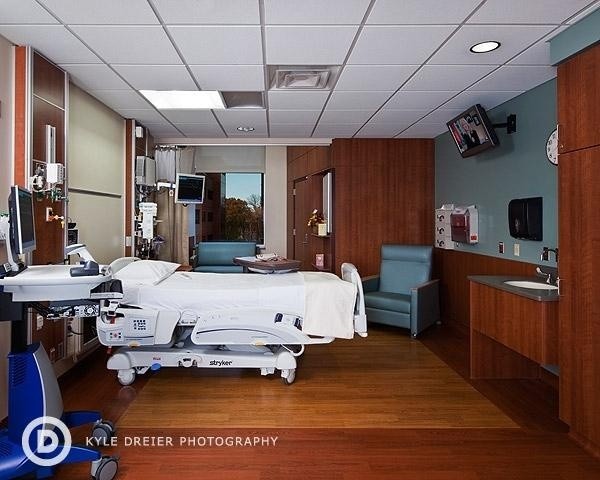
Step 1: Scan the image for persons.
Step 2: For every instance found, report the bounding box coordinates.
[459,118,481,150]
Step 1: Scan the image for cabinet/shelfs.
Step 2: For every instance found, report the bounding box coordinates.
[309,232,333,273]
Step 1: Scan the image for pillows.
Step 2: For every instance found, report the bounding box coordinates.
[112,259,183,288]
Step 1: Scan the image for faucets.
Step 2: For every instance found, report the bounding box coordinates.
[541,249,559,262]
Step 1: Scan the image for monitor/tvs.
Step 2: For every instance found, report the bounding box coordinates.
[0,186,36,277]
[446,103,500,158]
[175,173,205,204]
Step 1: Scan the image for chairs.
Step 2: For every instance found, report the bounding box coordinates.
[359,242,443,339]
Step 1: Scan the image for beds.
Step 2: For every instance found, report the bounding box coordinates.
[92,257,368,385]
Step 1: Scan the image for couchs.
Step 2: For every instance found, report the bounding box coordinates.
[188,241,262,274]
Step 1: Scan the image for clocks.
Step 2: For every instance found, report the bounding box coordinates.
[545,126,559,166]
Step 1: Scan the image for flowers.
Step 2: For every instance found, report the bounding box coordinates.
[307,208,326,227]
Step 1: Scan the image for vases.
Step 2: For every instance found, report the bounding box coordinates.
[312,224,318,234]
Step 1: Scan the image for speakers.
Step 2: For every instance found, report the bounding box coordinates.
[322,172,333,234]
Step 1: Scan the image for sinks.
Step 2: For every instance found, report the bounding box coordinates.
[504,280,558,290]
